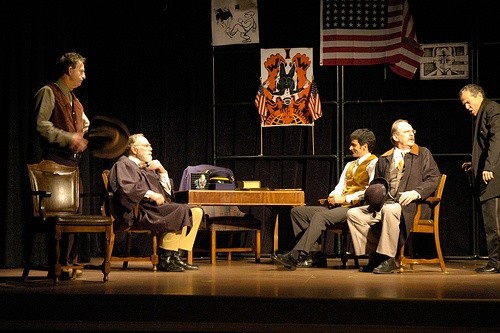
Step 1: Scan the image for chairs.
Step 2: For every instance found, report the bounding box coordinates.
[318,174,447,272]
[20,159,158,286]
[179,164,261,264]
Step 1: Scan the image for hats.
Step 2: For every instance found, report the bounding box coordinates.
[208,170,235,184]
[364,177,389,212]
[87,116,130,158]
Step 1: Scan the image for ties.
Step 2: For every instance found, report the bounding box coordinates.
[401,152,405,158]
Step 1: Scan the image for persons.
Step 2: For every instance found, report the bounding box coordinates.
[347,119,442,275]
[270,128,378,268]
[108,134,203,272]
[459,84,500,274]
[32,52,91,277]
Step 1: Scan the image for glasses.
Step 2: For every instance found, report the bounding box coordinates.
[395,130,416,135]
[130,144,152,148]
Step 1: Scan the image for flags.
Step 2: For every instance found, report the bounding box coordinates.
[321,0,425,80]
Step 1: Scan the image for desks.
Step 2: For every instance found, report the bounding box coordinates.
[173,190,305,264]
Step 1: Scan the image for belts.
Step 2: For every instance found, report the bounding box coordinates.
[68,153,81,159]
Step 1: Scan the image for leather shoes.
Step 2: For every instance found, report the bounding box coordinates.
[156,248,185,272]
[171,250,198,270]
[296,252,327,268]
[359,253,381,272]
[372,256,398,274]
[475,264,497,273]
[270,254,297,270]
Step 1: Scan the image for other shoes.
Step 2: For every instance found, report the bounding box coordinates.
[47,266,83,277]
